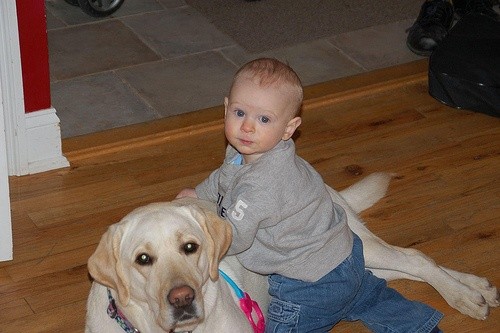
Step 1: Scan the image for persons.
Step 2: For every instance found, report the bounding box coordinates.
[173,57,444,333]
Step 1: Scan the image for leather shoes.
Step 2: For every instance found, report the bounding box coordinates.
[406,0,454,56]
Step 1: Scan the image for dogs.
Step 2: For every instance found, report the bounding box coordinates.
[85,170,500,333]
